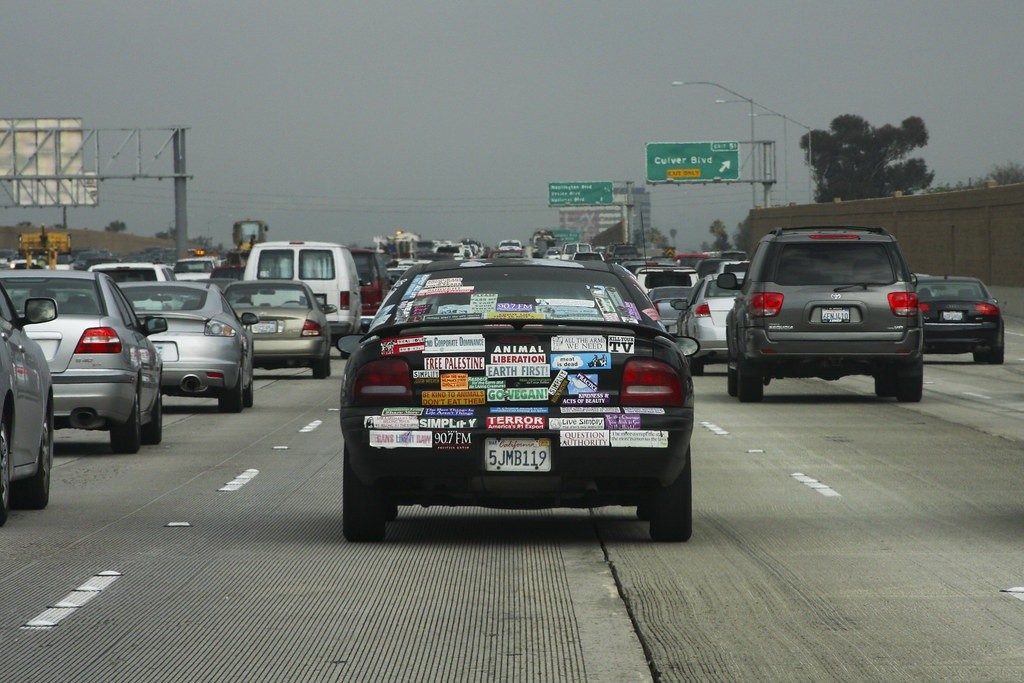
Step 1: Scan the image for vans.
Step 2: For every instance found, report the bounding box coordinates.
[346,250,394,336]
[636,265,699,292]
[243,242,361,358]
[695,258,742,279]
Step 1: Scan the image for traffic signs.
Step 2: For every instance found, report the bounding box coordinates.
[645,142,740,183]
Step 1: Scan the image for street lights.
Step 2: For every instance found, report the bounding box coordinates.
[671,80,811,207]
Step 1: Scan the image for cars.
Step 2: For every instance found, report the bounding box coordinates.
[1,269,168,452]
[222,280,337,378]
[675,271,747,376]
[0,282,54,522]
[3,216,269,280]
[119,281,260,413]
[718,259,752,271]
[912,276,1003,364]
[338,256,701,542]
[645,285,697,338]
[718,251,747,262]
[898,273,937,295]
[372,230,717,289]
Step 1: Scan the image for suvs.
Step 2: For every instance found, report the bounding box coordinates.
[717,224,925,402]
